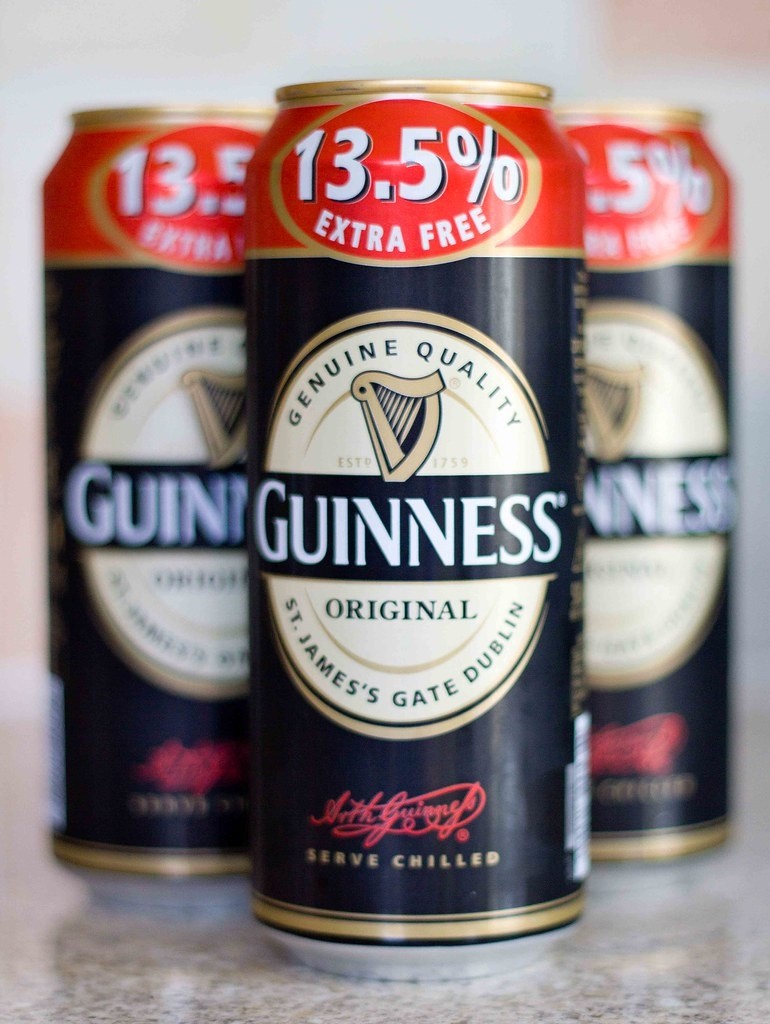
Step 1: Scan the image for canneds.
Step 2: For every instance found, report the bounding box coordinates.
[38,105,280,879]
[539,105,735,862]
[242,77,594,944]
